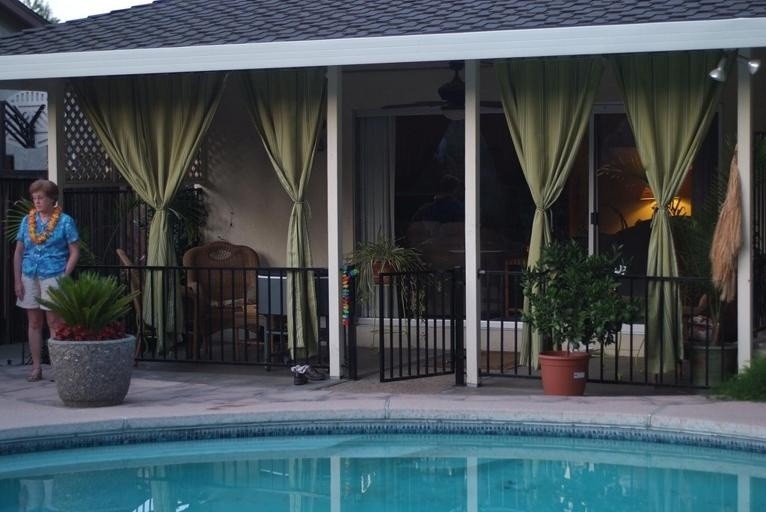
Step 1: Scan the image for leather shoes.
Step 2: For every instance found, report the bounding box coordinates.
[294,365,325,385]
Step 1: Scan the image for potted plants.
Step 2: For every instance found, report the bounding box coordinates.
[344,227,440,326]
[31,268,141,407]
[522,236,628,397]
[593,157,737,395]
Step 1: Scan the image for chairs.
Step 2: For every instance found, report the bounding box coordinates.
[183,240,261,367]
[114,249,206,366]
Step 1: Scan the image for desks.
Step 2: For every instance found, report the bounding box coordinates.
[253,262,355,374]
[231,310,287,367]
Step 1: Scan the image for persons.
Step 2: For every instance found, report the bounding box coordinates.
[684,265,716,341]
[12,179,80,382]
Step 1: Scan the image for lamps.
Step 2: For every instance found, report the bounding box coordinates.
[733,48,762,77]
[709,48,729,81]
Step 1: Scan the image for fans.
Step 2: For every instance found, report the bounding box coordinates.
[377,60,506,117]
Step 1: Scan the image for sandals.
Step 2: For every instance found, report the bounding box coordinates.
[27,368,42,382]
